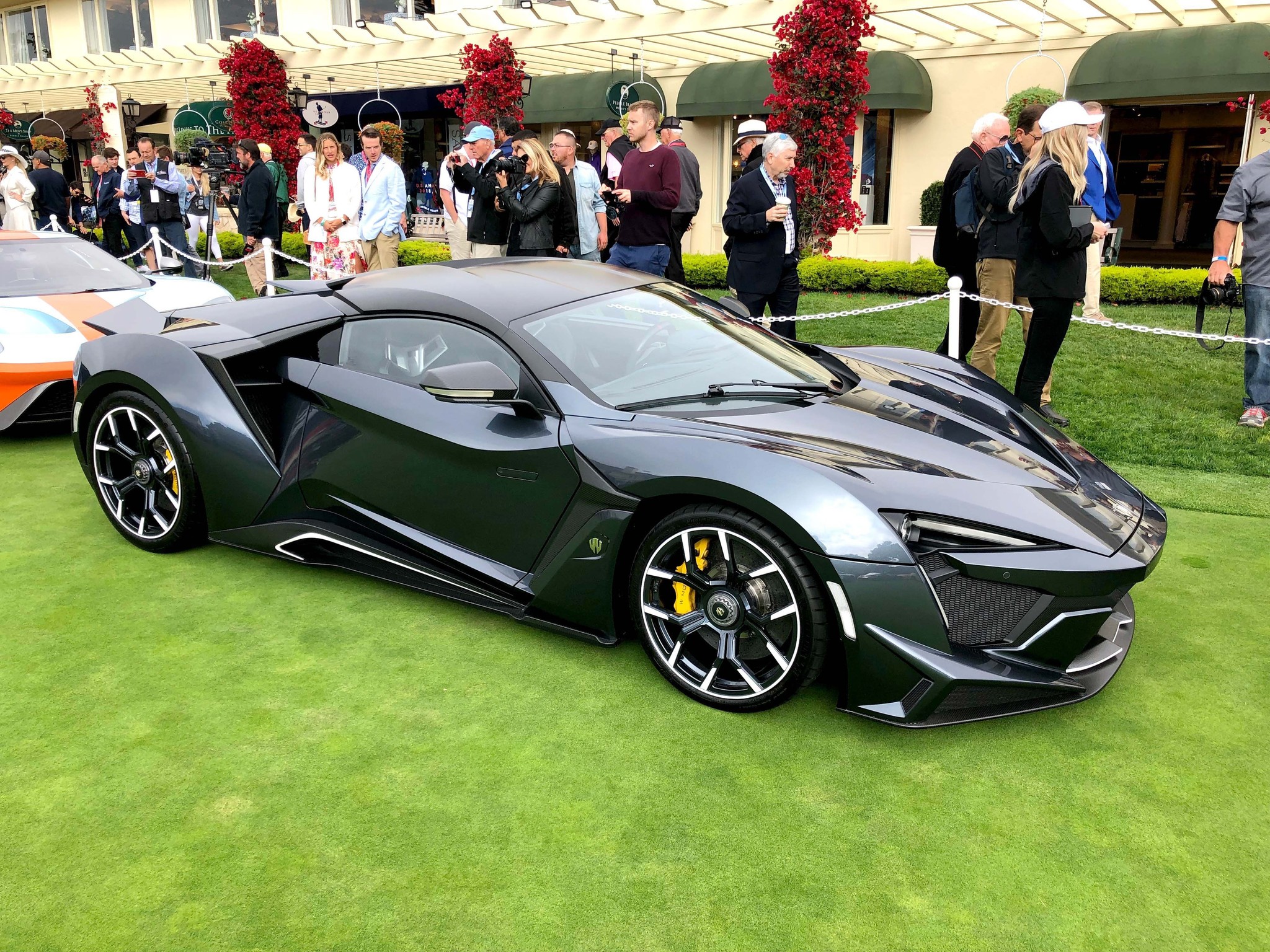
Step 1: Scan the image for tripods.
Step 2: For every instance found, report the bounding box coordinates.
[201,173,240,284]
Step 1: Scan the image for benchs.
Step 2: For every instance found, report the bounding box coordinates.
[406,214,449,245]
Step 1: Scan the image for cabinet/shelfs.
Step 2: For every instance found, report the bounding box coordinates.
[1217,164,1239,196]
[1119,160,1169,198]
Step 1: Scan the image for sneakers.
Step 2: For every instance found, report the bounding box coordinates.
[1237,406,1270,427]
[136,265,150,272]
[1090,312,1113,322]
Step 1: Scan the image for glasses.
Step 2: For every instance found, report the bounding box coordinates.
[295,143,310,148]
[737,141,748,150]
[521,154,529,162]
[986,131,1042,144]
[549,144,571,149]
[600,130,611,137]
[72,189,81,194]
[770,133,792,151]
[238,139,248,152]
[138,150,153,155]
[1,155,13,160]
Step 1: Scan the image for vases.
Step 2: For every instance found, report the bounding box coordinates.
[250,26,257,32]
[140,41,144,46]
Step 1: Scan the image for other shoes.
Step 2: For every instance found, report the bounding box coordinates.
[260,286,268,297]
[200,275,215,283]
[219,264,234,271]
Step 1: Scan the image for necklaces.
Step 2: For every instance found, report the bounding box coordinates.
[10,165,15,171]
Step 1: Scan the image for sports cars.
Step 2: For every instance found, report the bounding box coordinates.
[70,257,1170,733]
[0,229,244,434]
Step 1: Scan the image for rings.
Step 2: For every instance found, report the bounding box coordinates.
[777,214,780,219]
[13,196,15,197]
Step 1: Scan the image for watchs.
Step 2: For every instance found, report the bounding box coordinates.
[340,217,347,226]
[79,224,83,227]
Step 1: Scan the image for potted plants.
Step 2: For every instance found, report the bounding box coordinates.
[43,49,52,61]
[173,129,216,154]
[357,121,409,167]
[26,32,38,61]
[907,179,944,264]
[30,135,71,163]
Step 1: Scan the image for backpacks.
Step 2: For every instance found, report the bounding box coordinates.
[955,146,1012,237]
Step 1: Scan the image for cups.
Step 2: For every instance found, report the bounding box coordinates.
[775,197,791,220]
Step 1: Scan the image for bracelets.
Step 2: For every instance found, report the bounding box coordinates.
[1211,255,1226,264]
[320,218,326,226]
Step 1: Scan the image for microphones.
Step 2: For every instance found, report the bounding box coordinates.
[193,138,212,145]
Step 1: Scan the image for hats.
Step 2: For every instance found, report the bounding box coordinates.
[656,116,684,133]
[0,146,28,169]
[586,140,598,150]
[257,143,272,154]
[595,118,622,135]
[549,129,581,149]
[29,150,50,161]
[733,119,775,146]
[1038,100,1105,135]
[461,121,495,144]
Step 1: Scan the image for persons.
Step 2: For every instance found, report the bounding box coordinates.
[292,125,407,280]
[1207,150,1270,426]
[236,138,289,297]
[420,162,436,214]
[587,140,601,179]
[398,163,412,219]
[722,119,801,341]
[0,137,234,283]
[439,100,703,287]
[933,101,1122,427]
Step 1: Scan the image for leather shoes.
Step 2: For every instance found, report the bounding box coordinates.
[1040,404,1070,427]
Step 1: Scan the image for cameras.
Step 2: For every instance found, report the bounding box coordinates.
[451,156,458,163]
[1201,274,1243,309]
[487,155,524,174]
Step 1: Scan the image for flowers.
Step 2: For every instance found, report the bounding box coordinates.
[245,10,257,27]
[133,29,146,43]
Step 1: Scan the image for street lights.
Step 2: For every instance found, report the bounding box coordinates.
[121,93,142,141]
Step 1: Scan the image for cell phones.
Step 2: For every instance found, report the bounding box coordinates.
[79,193,94,202]
[602,191,618,201]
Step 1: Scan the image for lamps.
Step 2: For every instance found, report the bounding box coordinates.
[287,81,308,112]
[2,104,14,119]
[513,70,533,110]
